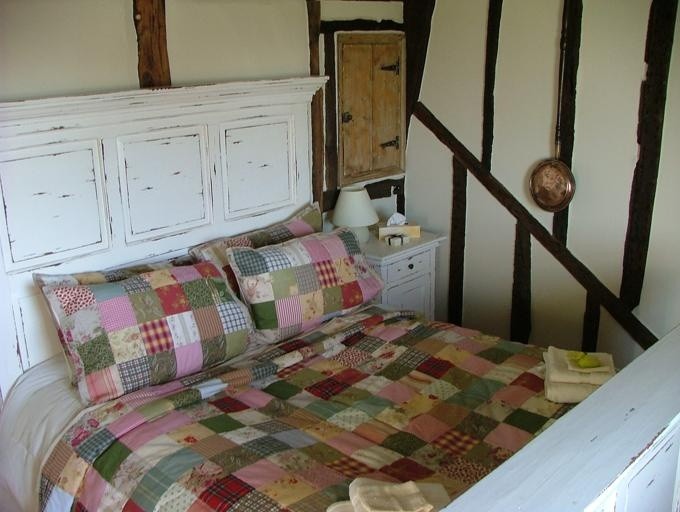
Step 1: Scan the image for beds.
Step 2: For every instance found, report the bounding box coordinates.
[0,299,678,511]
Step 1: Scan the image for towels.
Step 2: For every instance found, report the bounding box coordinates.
[325,477,450,512]
[542,343,616,404]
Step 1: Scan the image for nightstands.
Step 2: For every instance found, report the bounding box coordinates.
[350,217,449,320]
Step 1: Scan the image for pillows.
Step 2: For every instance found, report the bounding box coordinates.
[33,256,258,406]
[189,201,385,344]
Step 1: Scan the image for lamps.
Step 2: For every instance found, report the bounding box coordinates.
[333,185,380,242]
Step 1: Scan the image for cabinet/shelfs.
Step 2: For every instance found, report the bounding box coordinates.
[324,29,406,185]
